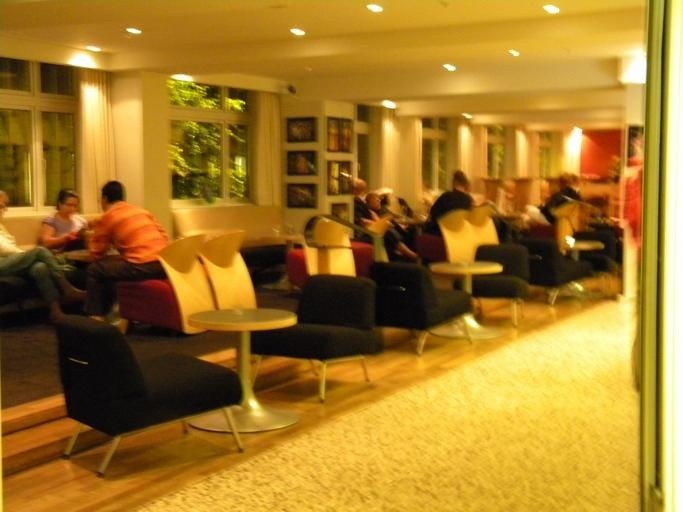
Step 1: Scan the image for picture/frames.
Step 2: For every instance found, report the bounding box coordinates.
[285,115,353,221]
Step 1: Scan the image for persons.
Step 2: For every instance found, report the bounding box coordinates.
[354,177,418,265]
[542,172,582,224]
[82,179,170,317]
[422,168,473,238]
[34,188,88,254]
[0,190,86,323]
[571,174,581,193]
